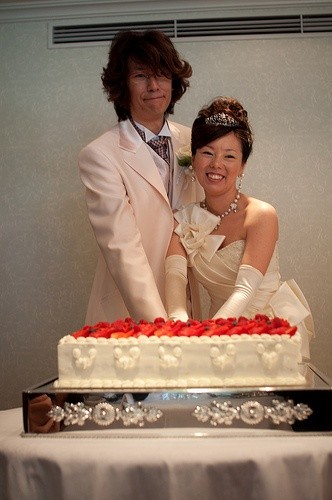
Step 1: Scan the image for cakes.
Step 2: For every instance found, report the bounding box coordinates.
[53,313,307,387]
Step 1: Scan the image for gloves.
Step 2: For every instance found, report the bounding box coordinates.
[213,264,262,320]
[164,254,188,323]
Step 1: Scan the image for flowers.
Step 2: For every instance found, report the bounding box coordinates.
[177,144,197,184]
[176,204,226,262]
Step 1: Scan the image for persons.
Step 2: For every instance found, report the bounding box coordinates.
[166,97,316,359]
[78,28,207,327]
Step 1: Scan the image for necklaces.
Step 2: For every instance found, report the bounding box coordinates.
[202,197,239,230]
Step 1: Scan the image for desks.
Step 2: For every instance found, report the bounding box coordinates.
[0,408,332,499]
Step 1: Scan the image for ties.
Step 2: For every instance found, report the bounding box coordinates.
[129,116,171,165]
